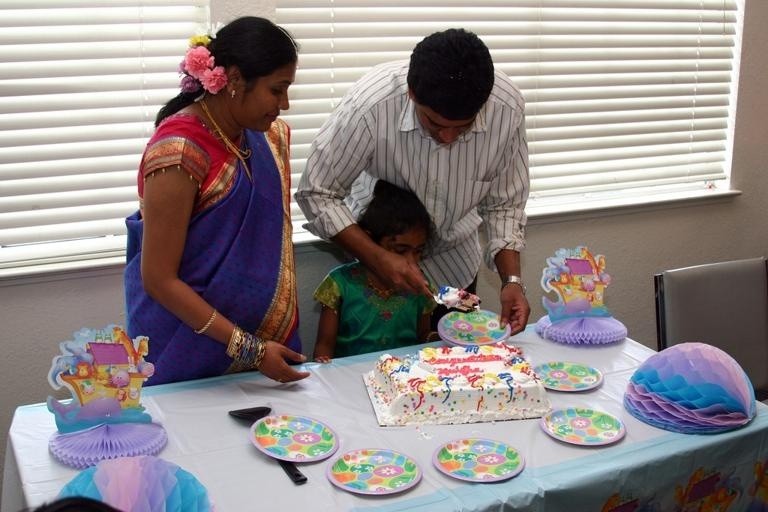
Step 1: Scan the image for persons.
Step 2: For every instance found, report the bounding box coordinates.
[294,29,531,347]
[122,16,310,386]
[314,180,441,363]
[30,496,122,512]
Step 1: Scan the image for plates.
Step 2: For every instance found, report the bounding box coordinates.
[438,310,511,349]
[540,407,626,446]
[534,362,604,391]
[326,448,422,495]
[251,415,339,461]
[433,438,526,483]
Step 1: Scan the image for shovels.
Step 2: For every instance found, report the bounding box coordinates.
[428,286,482,313]
[228,406,308,484]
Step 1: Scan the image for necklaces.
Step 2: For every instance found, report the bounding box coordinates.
[200,99,254,187]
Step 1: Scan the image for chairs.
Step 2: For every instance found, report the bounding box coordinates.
[652,256,767,407]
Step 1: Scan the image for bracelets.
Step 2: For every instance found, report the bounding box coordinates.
[195,309,218,335]
[502,274,527,295]
[226,323,265,370]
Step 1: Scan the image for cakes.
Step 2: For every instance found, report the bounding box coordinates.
[433,284,482,313]
[372,341,551,427]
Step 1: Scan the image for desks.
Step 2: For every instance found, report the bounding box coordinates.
[8,323,767,512]
